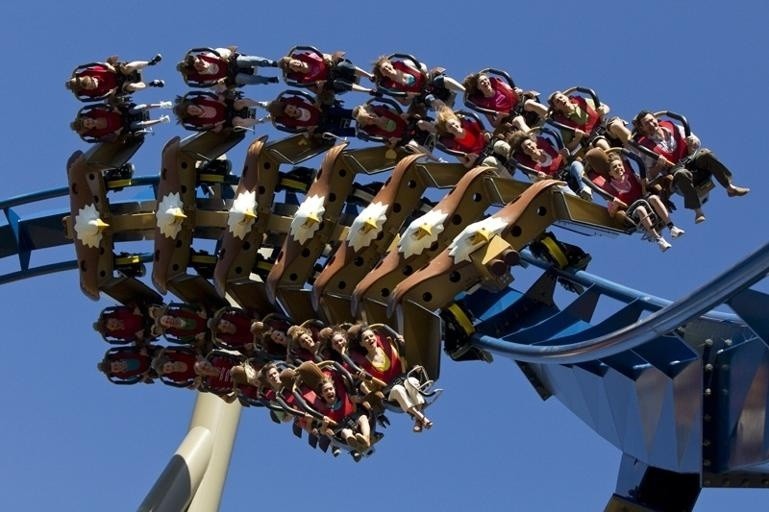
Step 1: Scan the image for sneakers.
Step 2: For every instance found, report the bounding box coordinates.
[273,60,278,67]
[333,433,368,462]
[671,226,684,238]
[260,101,268,108]
[160,101,172,109]
[695,211,705,223]
[727,186,751,197]
[152,54,162,65]
[153,79,164,87]
[369,88,384,97]
[160,115,170,123]
[580,187,593,202]
[260,113,271,124]
[369,74,375,83]
[270,77,280,84]
[657,236,672,252]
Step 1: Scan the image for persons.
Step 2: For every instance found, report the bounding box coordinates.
[436,72,549,180]
[187,51,283,91]
[195,305,433,449]
[607,110,750,252]
[81,101,174,133]
[288,55,381,99]
[105,304,215,390]
[350,104,448,164]
[513,91,631,201]
[75,53,164,95]
[371,55,467,114]
[173,95,277,134]
[279,101,361,140]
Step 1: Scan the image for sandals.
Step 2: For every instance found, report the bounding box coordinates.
[413,416,433,432]
